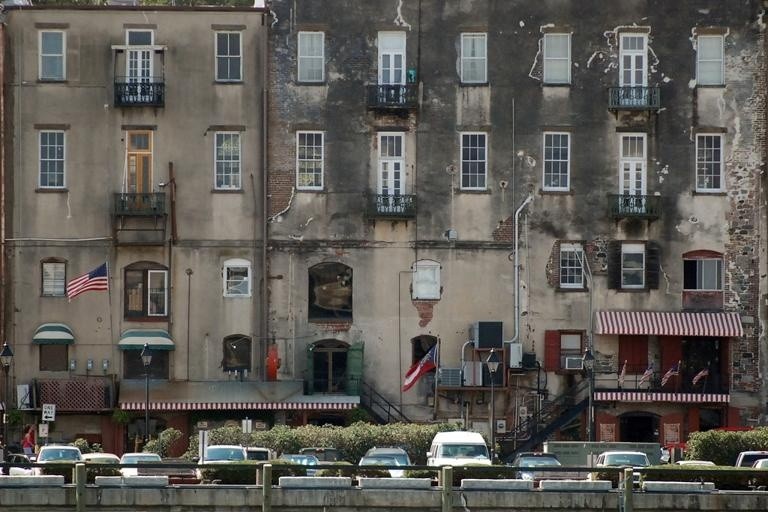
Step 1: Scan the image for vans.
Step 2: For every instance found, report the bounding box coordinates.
[736,451,768,468]
[596,451,652,488]
[426,431,492,479]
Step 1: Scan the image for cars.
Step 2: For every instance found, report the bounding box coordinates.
[675,461,717,466]
[510,451,562,481]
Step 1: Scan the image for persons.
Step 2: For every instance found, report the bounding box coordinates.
[22,423,36,454]
[660,443,673,463]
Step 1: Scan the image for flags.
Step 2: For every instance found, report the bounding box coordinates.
[638,365,654,385]
[65,260,108,301]
[617,362,626,386]
[660,362,680,386]
[692,366,710,385]
[400,343,435,391]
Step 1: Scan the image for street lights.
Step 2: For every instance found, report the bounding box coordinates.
[141,343,152,445]
[0,341,14,456]
[486,347,501,460]
[582,347,595,442]
